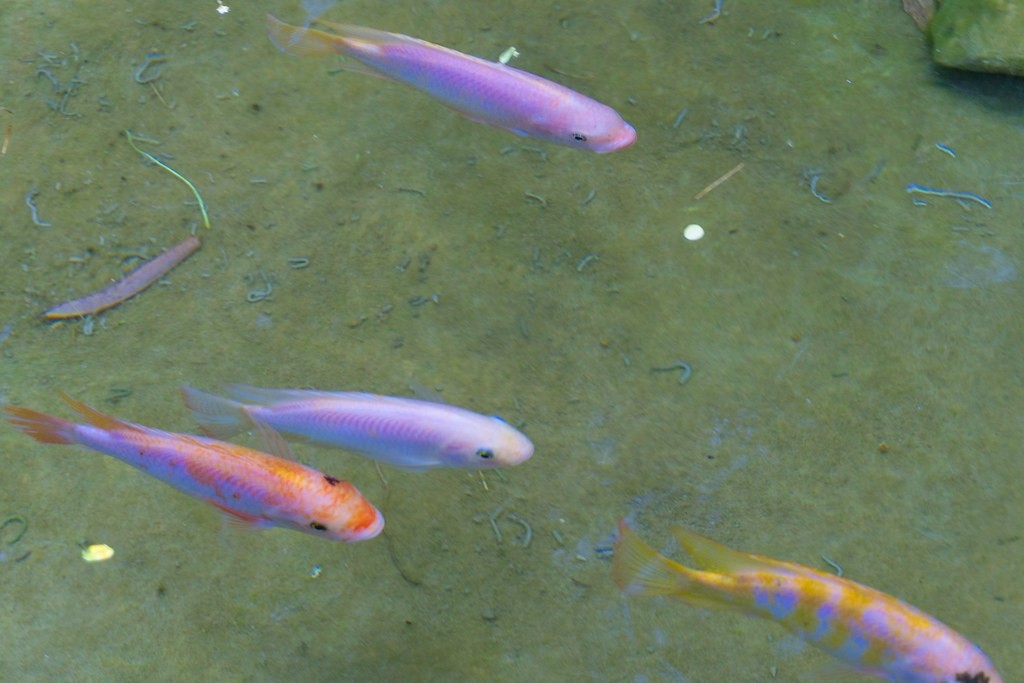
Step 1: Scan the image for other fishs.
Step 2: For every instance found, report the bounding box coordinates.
[611,514,1002,683]
[2,379,534,544]
[263,13,637,154]
